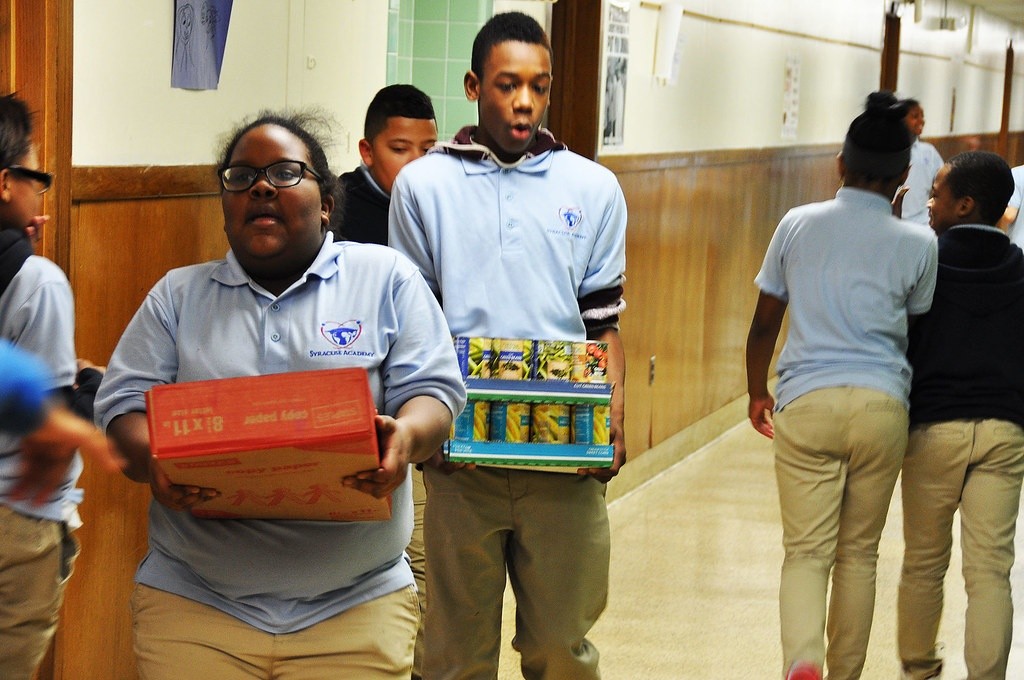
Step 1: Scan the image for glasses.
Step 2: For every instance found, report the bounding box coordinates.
[218,159,324,193]
[6,165,51,195]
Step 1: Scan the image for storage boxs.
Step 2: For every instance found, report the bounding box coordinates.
[144,367,392,522]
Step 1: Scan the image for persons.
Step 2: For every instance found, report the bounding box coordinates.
[0,339,126,475]
[1000,165,1024,255]
[388,12,628,680]
[744,110,938,680]
[89,116,468,680]
[333,84,437,680]
[0,93,106,680]
[892,100,944,225]
[895,150,1024,680]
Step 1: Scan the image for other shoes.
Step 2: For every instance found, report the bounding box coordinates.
[787,662,821,680]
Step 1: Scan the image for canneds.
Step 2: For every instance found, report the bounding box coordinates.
[468,337,610,445]
